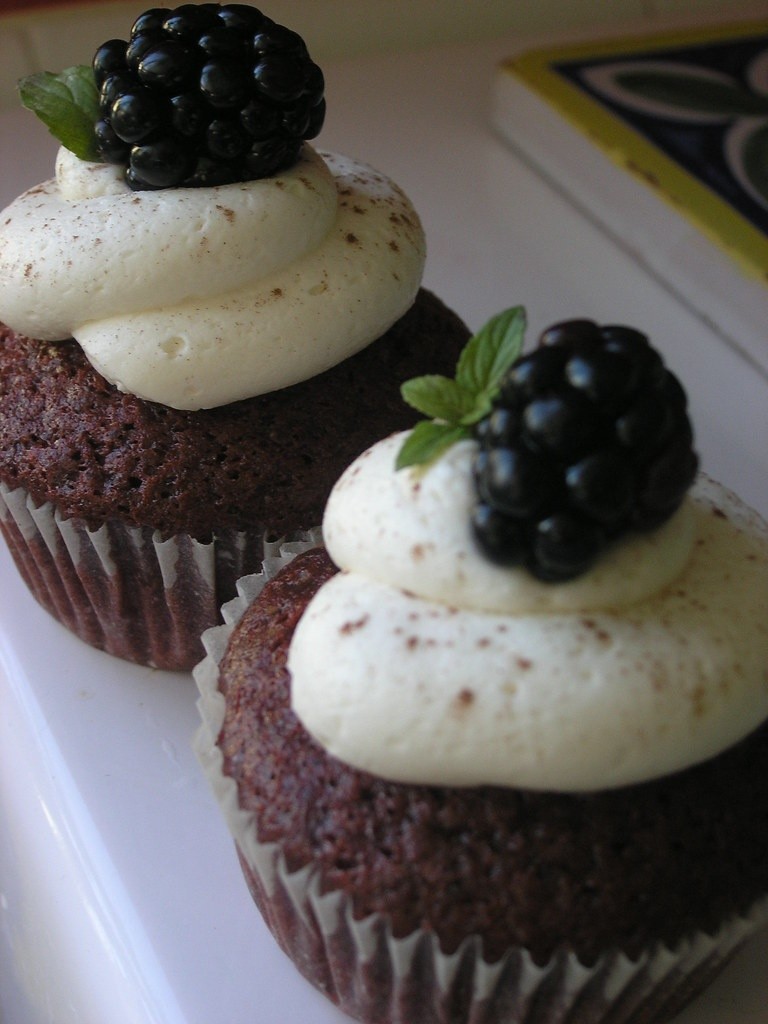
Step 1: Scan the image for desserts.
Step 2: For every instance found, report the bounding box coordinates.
[0,0,476,671]
[188,305,768,1024]
